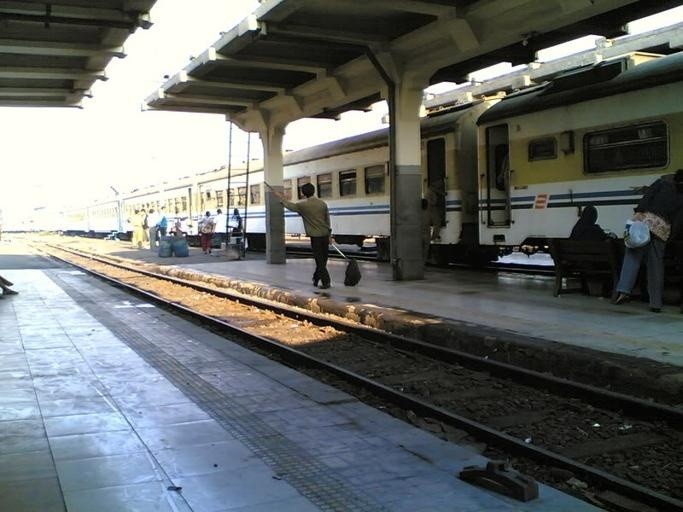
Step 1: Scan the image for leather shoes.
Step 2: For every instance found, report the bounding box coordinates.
[312,277,331,289]
[607,291,631,304]
[648,303,661,313]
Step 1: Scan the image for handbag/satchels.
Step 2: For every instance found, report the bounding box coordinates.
[623,219,652,249]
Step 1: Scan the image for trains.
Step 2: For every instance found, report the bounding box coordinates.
[0,50,683,269]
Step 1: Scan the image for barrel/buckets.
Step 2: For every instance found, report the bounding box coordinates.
[159,237,172,256]
[173,237,189,257]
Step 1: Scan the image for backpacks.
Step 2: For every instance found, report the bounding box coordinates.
[160,217,167,229]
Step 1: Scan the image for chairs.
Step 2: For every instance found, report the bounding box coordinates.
[543,239,598,298]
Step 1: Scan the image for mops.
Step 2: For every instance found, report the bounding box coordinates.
[264,180,362,287]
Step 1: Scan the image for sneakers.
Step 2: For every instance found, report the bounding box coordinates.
[2,278,19,295]
[200,252,214,257]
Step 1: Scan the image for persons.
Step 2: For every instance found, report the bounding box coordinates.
[565,205,619,297]
[612,166,683,311]
[424,178,447,245]
[127,205,243,256]
[272,183,335,289]
